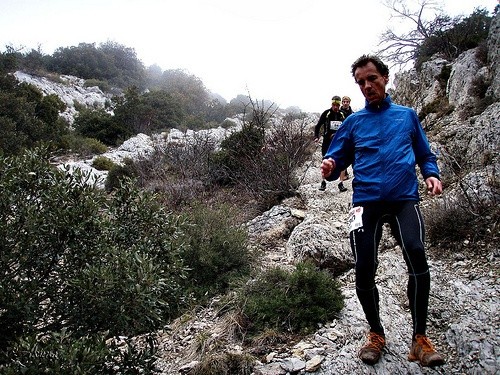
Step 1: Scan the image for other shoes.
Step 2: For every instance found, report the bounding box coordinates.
[338,182,347,192]
[344,175,349,180]
[319,180,326,190]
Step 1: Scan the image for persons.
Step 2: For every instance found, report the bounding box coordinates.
[339,96,355,180]
[314,96,348,192]
[321,53,445,365]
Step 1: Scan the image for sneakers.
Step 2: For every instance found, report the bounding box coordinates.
[358,329,386,365]
[408,335,446,367]
